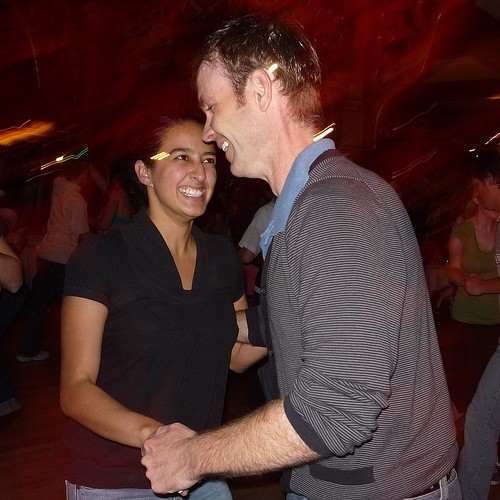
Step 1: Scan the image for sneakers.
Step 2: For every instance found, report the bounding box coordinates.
[15,350,50,362]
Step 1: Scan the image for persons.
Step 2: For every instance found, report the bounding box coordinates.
[150,12,463,500]
[0,157,135,362]
[429,150,500,500]
[60,114,269,500]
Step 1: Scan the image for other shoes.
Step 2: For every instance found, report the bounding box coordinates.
[491,463,500,486]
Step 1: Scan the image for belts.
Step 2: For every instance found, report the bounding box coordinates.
[409,470,453,498]
